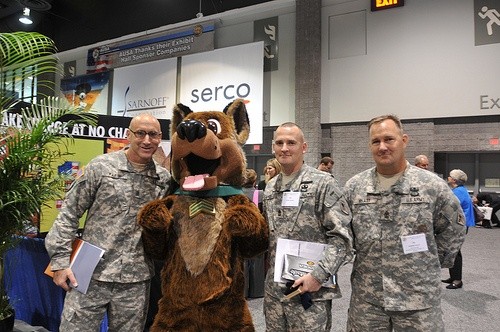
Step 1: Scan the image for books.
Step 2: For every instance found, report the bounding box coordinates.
[44,237,105,295]
[274,239,337,290]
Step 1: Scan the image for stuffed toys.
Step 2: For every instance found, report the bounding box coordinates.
[137,99,269,332]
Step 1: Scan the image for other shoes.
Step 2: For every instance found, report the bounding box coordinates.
[447,281,463,289]
[440,277,451,283]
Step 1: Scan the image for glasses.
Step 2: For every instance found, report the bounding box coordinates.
[127,126,162,141]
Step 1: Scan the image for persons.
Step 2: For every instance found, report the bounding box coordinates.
[343,115,475,332]
[240,122,353,332]
[46,115,179,332]
[476,192,500,229]
[15,212,38,237]
